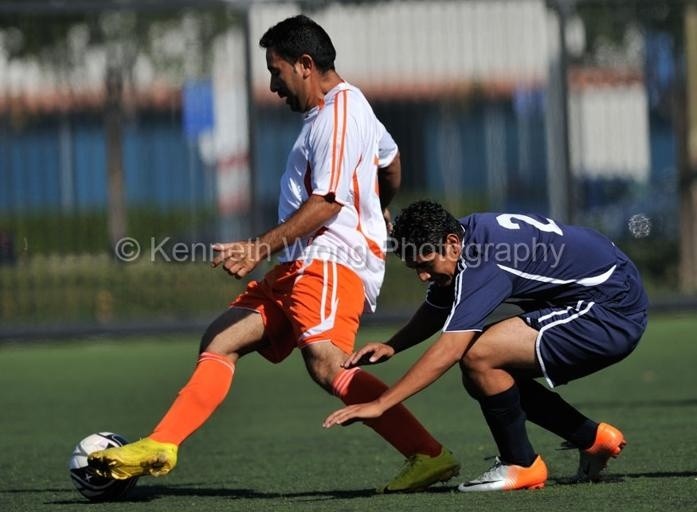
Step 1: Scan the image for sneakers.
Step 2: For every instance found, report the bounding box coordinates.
[457,451,546,494]
[86,435,180,481]
[383,448,461,494]
[575,421,625,486]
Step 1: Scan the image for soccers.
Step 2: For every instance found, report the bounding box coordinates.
[70,432,139,501]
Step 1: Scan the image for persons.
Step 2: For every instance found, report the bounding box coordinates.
[87,15,463,495]
[323,199,648,493]
